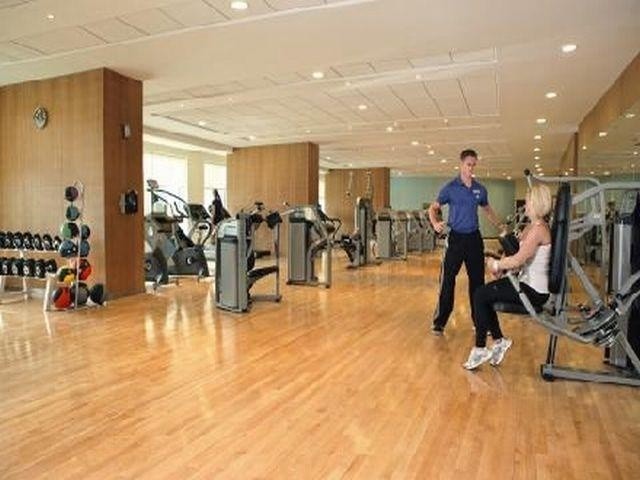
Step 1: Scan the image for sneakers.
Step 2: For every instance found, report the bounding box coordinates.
[462,345,493,371]
[431,324,444,336]
[487,337,514,367]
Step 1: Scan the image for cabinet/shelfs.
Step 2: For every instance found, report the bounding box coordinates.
[0,248,76,300]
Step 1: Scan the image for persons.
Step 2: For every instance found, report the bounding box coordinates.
[428,149,507,337]
[462,185,553,371]
[210,189,223,244]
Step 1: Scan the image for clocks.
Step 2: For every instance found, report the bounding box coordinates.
[33,106,47,127]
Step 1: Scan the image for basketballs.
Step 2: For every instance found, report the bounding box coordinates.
[52,186,109,309]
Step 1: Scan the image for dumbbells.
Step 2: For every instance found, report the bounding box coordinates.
[0,257,57,277]
[0,231,62,251]
